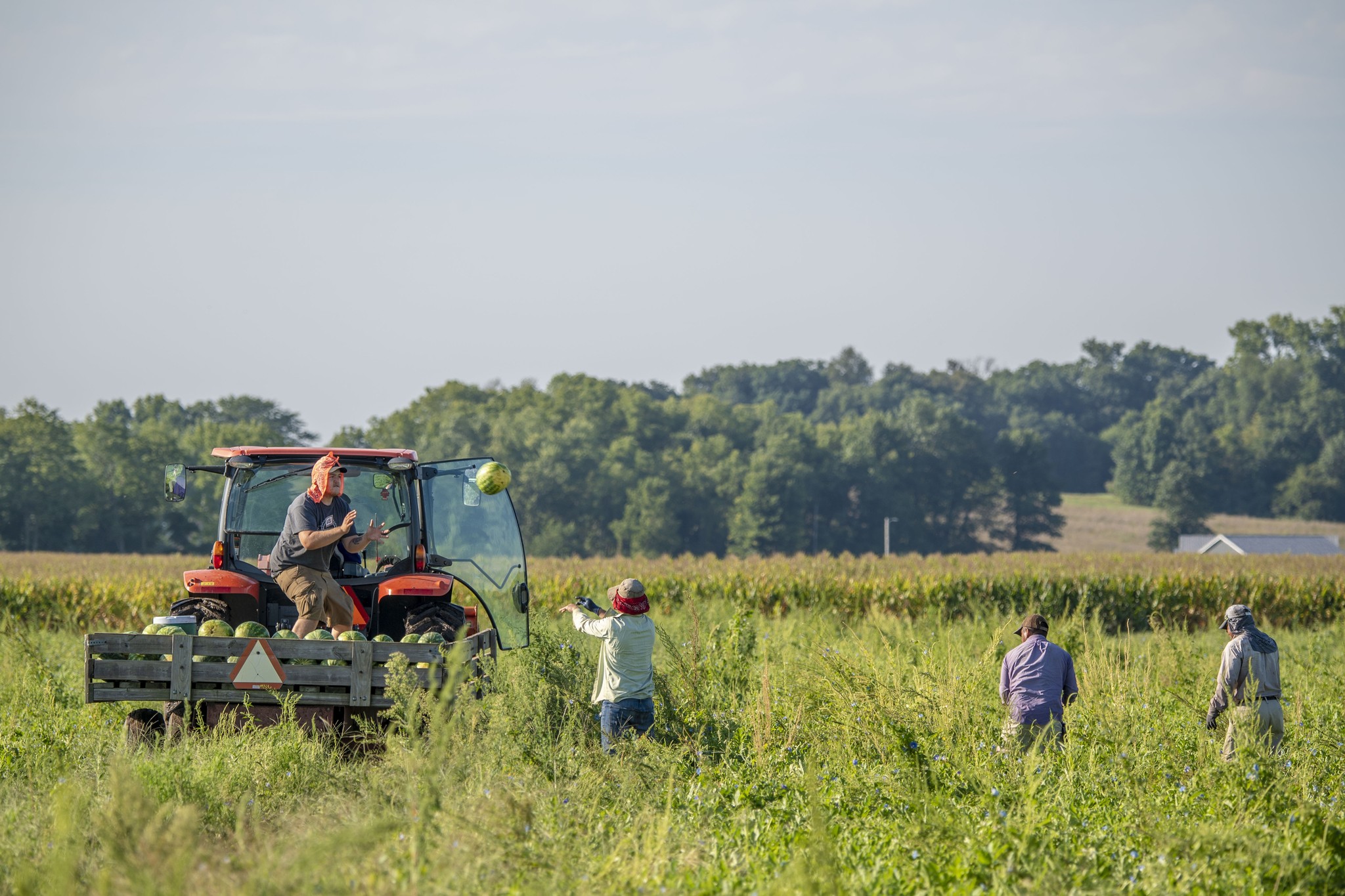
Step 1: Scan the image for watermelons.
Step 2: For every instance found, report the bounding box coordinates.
[476,462,512,495]
[95,623,446,682]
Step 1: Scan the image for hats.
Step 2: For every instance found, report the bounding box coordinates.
[1013,613,1049,637]
[328,463,347,473]
[1218,604,1251,629]
[608,578,644,604]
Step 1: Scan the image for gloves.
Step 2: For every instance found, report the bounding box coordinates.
[575,596,602,616]
[1206,714,1217,730]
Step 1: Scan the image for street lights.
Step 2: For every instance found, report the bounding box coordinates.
[883,516,899,559]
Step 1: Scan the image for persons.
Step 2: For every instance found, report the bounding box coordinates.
[1206,604,1284,759]
[999,613,1079,755]
[270,451,390,639]
[559,578,655,755]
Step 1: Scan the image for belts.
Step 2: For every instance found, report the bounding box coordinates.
[1238,696,1277,703]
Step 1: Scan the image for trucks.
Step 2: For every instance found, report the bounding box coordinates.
[78,440,532,770]
